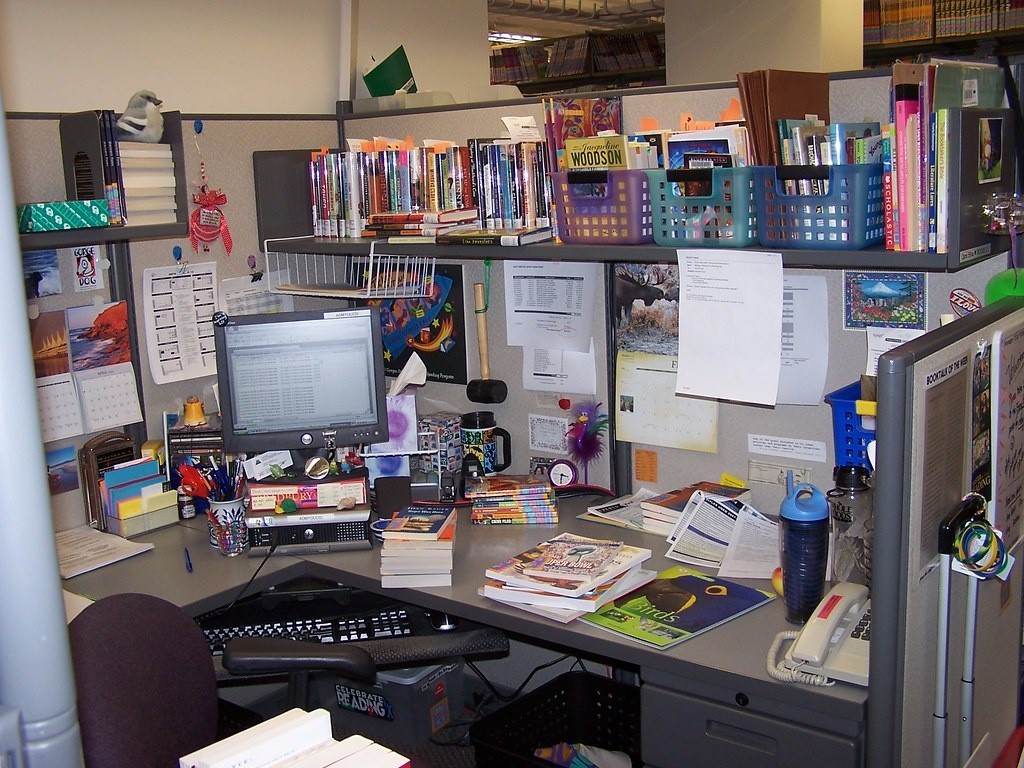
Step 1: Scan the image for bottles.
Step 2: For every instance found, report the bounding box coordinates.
[207,496,247,549]
[459,453,485,501]
[183,395,206,427]
[780,482,830,624]
[178,486,196,520]
[829,466,875,592]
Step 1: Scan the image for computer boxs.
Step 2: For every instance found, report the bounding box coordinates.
[244,503,373,558]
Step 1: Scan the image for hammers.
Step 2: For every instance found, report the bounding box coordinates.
[466,283,508,404]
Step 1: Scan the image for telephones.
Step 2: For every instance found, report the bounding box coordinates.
[783,580,869,689]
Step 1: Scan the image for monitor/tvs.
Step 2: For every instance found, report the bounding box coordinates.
[215,307,390,483]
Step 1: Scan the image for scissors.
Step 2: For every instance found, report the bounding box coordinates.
[179,464,212,498]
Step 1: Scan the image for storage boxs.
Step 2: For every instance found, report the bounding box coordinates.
[546,172,656,245]
[642,167,757,248]
[748,164,888,250]
[408,411,463,476]
[16,198,110,232]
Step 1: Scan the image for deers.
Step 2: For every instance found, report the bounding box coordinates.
[613,267,670,330]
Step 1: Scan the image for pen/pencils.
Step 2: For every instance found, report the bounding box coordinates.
[184,546,193,573]
[189,452,244,502]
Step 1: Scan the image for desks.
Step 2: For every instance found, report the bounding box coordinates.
[55,479,871,768]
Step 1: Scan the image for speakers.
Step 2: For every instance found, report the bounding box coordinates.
[374,476,413,522]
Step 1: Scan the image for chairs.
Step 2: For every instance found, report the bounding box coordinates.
[67,592,413,768]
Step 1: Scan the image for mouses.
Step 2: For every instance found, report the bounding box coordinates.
[424,611,460,630]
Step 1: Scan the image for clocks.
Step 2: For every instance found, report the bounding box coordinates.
[548,460,579,485]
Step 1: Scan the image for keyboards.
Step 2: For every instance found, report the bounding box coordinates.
[203,609,413,655]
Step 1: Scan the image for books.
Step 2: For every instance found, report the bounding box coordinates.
[478,530,777,650]
[180,708,409,768]
[309,64,1024,248]
[169,411,224,470]
[465,473,559,526]
[863,0,1024,42]
[488,29,665,82]
[641,480,752,537]
[380,504,458,588]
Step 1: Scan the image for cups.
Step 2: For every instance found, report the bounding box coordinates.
[459,411,511,476]
[370,519,392,543]
[217,524,243,557]
